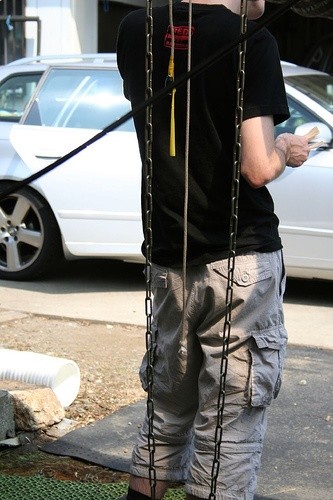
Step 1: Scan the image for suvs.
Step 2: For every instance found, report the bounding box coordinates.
[0,52,333,281]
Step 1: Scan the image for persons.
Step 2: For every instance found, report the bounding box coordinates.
[115,0,312,500]
